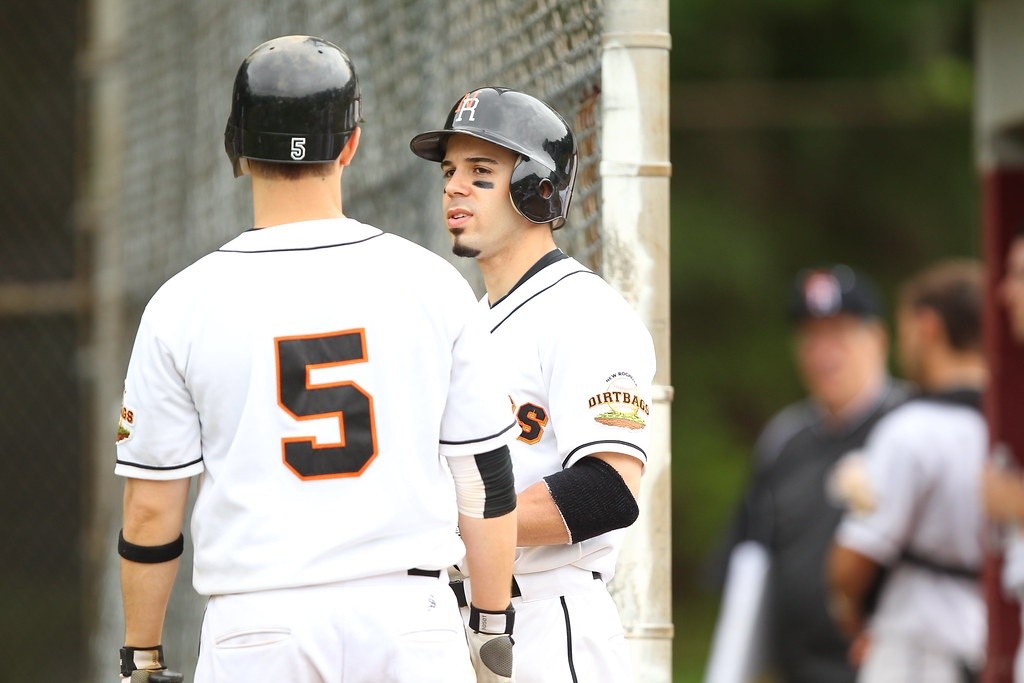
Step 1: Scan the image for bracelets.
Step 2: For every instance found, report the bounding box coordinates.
[540,457,641,545]
[119,528,186,565]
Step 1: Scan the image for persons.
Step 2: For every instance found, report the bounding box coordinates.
[698,228,1024,683]
[115,36,522,683]
[411,86,656,683]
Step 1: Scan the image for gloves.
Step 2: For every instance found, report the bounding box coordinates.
[465,603,515,683]
[119,645,183,683]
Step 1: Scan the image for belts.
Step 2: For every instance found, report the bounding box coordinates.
[449,571,601,606]
[408,568,442,578]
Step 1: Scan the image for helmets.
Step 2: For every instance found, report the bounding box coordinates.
[411,87,579,231]
[785,263,885,321]
[223,35,366,179]
